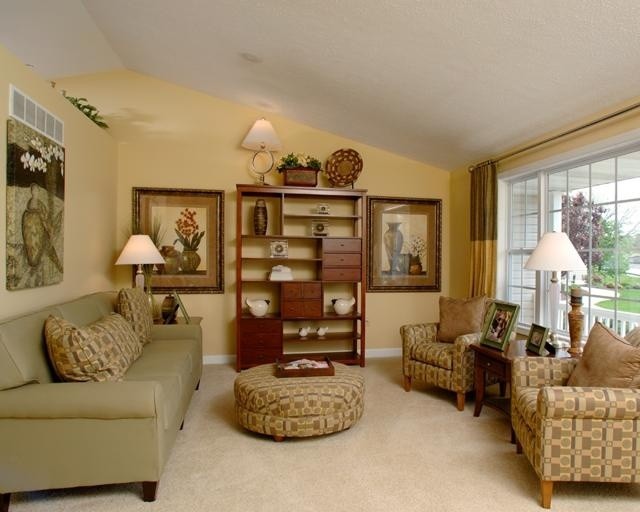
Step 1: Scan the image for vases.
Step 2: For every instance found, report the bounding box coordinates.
[153,245,181,274]
[179,250,201,272]
[282,169,317,188]
[382,221,404,274]
[409,260,422,275]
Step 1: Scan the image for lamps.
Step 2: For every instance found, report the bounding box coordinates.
[113,231,166,292]
[523,230,590,357]
[242,118,281,187]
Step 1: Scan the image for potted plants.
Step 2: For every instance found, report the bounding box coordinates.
[122,213,167,320]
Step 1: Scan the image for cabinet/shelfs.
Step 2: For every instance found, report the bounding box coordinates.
[232,184,368,372]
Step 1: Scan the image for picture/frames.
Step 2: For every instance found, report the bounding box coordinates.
[131,186,225,295]
[368,196,443,292]
[525,322,555,355]
[480,299,522,351]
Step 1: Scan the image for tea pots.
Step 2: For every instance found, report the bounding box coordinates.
[317,326,330,336]
[245,296,271,318]
[297,325,312,337]
[331,296,356,315]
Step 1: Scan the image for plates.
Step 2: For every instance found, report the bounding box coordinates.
[326,146,362,187]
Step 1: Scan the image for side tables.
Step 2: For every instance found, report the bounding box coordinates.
[469,338,585,444]
[154,314,203,326]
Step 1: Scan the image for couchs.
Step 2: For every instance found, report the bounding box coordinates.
[399,320,507,411]
[511,327,640,509]
[233,361,365,443]
[1,290,203,512]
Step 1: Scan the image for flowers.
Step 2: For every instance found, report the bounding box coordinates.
[173,207,206,250]
[278,151,326,171]
[404,234,427,262]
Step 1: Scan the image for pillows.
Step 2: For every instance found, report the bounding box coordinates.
[43,311,143,384]
[118,286,154,347]
[565,320,639,392]
[437,294,488,344]
